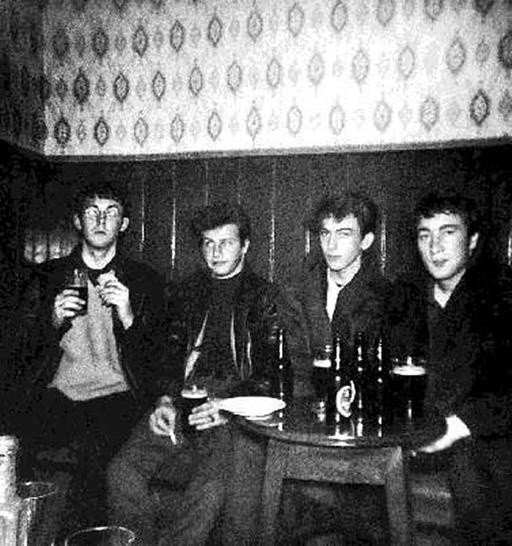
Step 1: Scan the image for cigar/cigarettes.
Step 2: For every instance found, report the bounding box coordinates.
[168,426,179,446]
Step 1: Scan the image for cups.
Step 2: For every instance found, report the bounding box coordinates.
[391,357,430,435]
[65,527,137,546]
[66,269,89,315]
[5,480,61,546]
[180,384,209,438]
[309,344,331,414]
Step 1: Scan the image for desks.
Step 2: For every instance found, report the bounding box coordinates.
[232,398,446,544]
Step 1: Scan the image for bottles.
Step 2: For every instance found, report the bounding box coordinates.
[330,331,387,437]
[0,436,27,546]
[273,330,293,401]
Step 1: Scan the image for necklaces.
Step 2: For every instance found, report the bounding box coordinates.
[326,269,358,290]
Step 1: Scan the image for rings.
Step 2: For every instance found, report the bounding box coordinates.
[207,413,216,426]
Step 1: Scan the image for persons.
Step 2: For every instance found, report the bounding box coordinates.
[383,184,512,546]
[2,179,168,546]
[108,199,288,544]
[285,188,393,546]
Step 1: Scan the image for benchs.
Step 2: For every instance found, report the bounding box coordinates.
[28,442,456,543]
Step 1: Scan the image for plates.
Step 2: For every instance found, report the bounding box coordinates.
[218,396,286,416]
